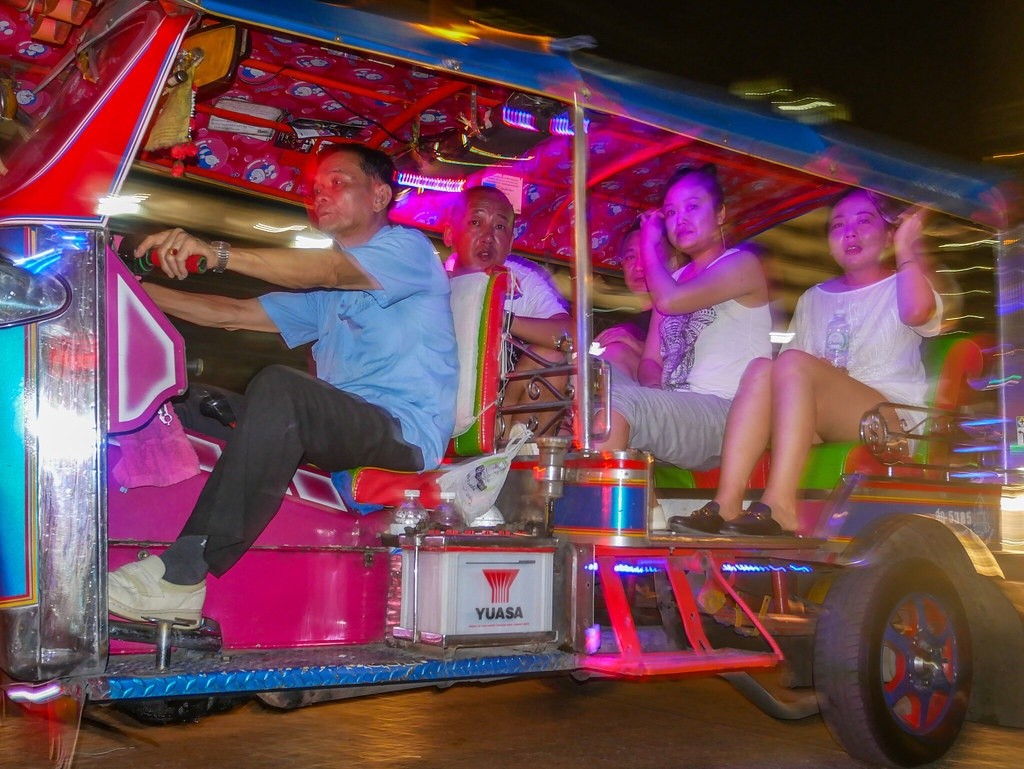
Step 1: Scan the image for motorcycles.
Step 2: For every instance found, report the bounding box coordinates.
[2,0,1024,768]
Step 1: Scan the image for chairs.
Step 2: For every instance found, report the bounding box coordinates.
[323,267,521,517]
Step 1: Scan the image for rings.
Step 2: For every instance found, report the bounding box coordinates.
[169,248,178,256]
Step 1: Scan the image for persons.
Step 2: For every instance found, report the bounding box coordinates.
[446,162,942,542]
[107,143,461,630]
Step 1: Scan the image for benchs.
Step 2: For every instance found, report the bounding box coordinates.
[643,327,986,500]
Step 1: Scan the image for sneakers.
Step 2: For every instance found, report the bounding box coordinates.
[109,553,208,628]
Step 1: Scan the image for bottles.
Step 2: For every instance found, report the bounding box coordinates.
[535,438,571,498]
[824,309,851,372]
[431,492,464,528]
[383,489,429,649]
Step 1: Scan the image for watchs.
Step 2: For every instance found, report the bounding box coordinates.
[503,309,515,334]
[209,240,232,274]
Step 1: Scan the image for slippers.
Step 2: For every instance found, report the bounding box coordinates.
[726,500,799,535]
[669,504,721,533]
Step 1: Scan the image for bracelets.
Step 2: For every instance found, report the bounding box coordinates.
[895,260,916,270]
